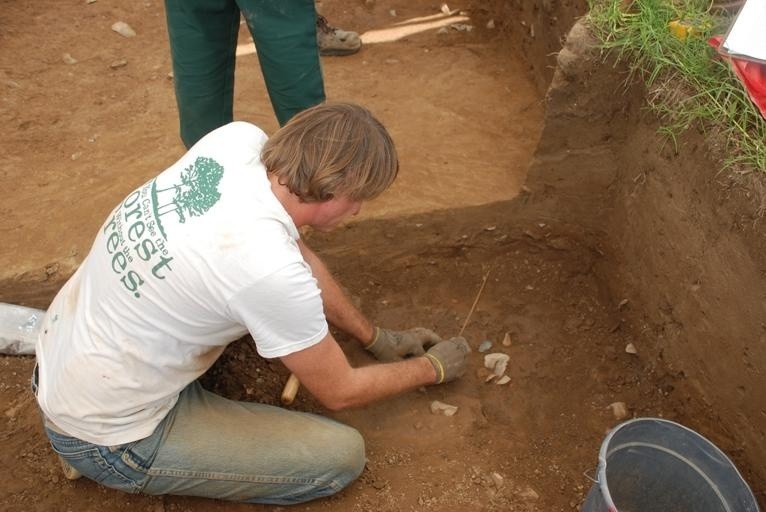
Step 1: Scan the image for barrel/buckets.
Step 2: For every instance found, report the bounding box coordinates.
[583,418,761,512]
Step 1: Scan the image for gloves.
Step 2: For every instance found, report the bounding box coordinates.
[366,325,442,363]
[424,336,473,387]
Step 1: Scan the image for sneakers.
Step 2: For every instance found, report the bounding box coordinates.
[315,12,361,58]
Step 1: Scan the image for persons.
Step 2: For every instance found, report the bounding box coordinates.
[159,2,333,155]
[27,99,474,511]
